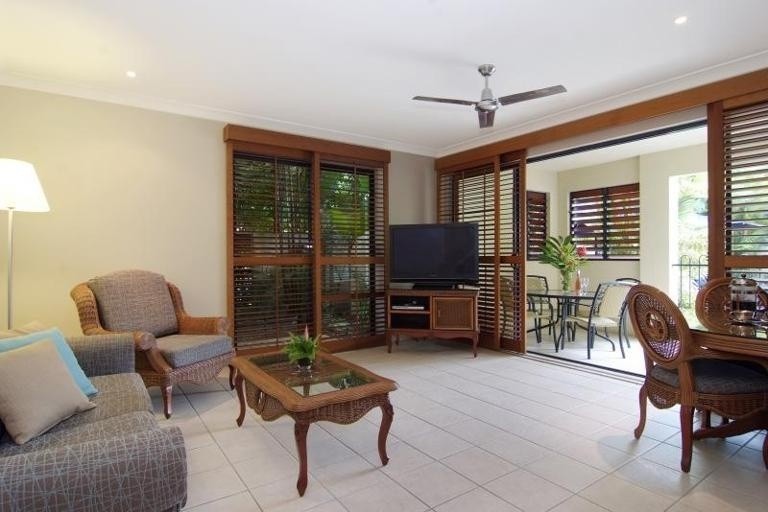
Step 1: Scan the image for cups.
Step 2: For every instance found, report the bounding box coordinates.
[581,278,589,295]
[728,310,753,324]
[754,298,766,323]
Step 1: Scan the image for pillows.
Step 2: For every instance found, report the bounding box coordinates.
[0,328,97,398]
[0,337,95,445]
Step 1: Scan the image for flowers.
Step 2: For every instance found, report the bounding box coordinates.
[538,235,587,270]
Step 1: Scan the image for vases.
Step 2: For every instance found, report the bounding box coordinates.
[560,271,573,293]
[297,364,312,371]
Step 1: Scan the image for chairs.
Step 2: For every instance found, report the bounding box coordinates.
[695,277,768,332]
[627,284,768,474]
[71,270,237,420]
[526,275,558,353]
[557,277,642,359]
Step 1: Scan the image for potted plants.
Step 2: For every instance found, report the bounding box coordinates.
[286,328,321,370]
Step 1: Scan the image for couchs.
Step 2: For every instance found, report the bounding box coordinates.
[0,333,188,512]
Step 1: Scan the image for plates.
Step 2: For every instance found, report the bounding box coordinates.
[725,318,766,326]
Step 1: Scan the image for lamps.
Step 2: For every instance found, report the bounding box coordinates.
[0,157,49,330]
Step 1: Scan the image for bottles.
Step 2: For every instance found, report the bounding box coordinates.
[576,270,581,295]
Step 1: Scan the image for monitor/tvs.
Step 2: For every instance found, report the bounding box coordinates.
[389,222,479,291]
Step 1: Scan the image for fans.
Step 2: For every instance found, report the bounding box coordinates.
[411,64,567,129]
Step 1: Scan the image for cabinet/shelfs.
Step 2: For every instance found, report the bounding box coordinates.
[385,289,479,358]
